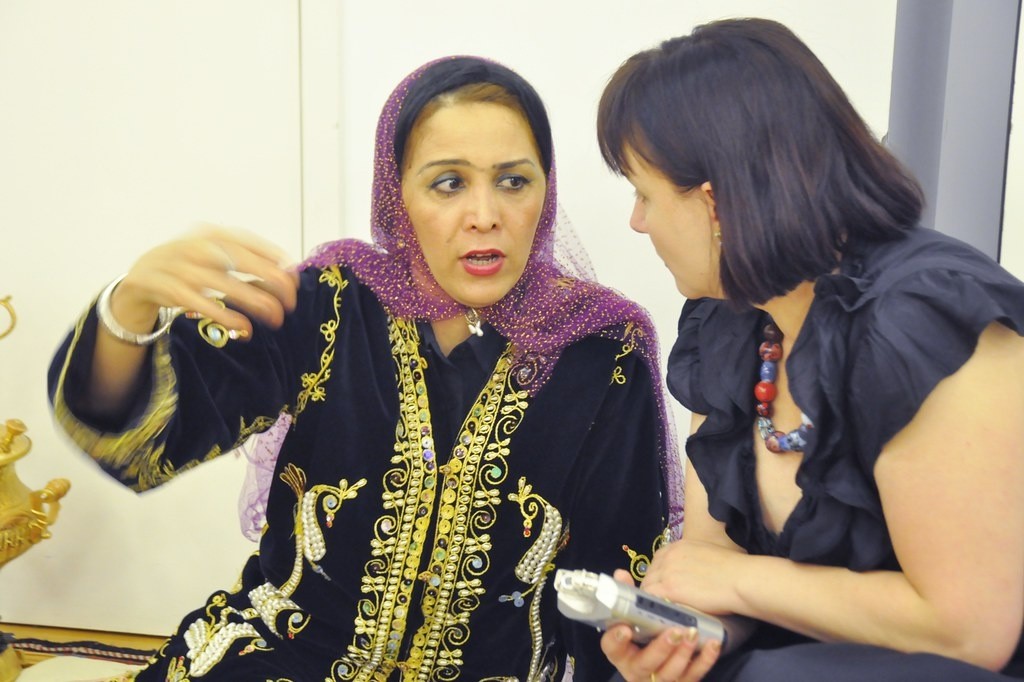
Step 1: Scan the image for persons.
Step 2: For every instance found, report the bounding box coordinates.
[47,55,684,682]
[597,19,1024,682]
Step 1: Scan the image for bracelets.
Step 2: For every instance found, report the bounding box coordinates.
[97,273,172,344]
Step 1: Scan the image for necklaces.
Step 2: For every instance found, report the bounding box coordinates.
[754,324,814,453]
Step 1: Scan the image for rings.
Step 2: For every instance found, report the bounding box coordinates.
[651,672,656,682]
